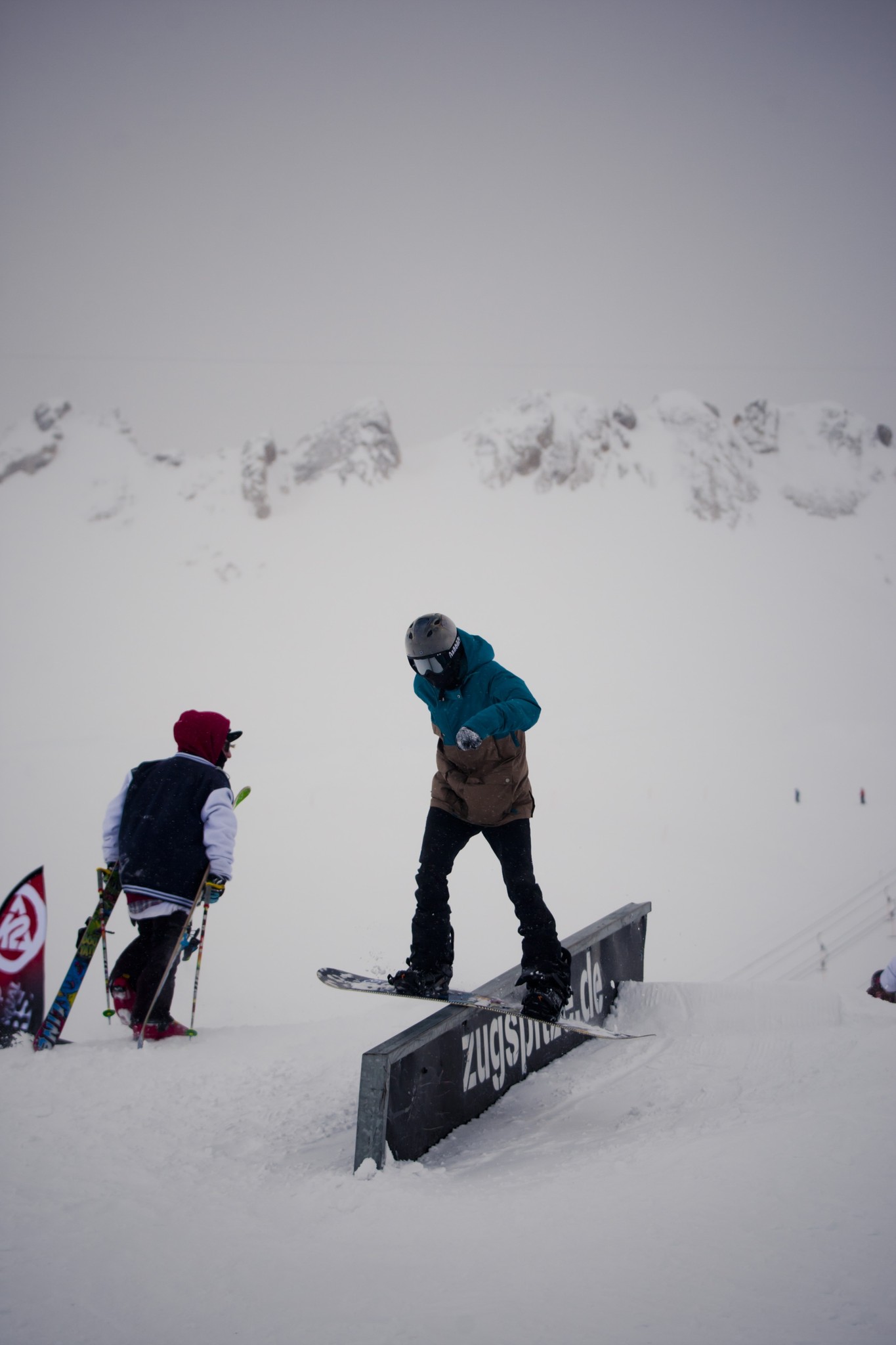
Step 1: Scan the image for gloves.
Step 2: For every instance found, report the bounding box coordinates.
[103,862,114,885]
[455,727,483,751]
[202,873,226,902]
[868,969,896,1003]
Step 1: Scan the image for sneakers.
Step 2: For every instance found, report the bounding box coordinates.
[109,976,138,1024]
[134,1014,193,1042]
[521,977,566,1022]
[387,964,455,1000]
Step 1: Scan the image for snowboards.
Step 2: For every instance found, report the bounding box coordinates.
[316,967,656,1039]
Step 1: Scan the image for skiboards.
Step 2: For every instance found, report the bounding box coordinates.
[32,786,250,1051]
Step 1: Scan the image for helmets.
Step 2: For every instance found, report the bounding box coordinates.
[403,613,458,659]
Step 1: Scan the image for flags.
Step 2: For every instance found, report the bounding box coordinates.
[0,865,48,1051]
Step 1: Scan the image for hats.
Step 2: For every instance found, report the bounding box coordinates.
[224,728,244,745]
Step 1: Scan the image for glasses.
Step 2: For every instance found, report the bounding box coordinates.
[409,652,449,676]
[223,740,230,751]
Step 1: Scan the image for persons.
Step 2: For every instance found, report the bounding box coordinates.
[101,707,246,1042]
[392,612,575,1026]
